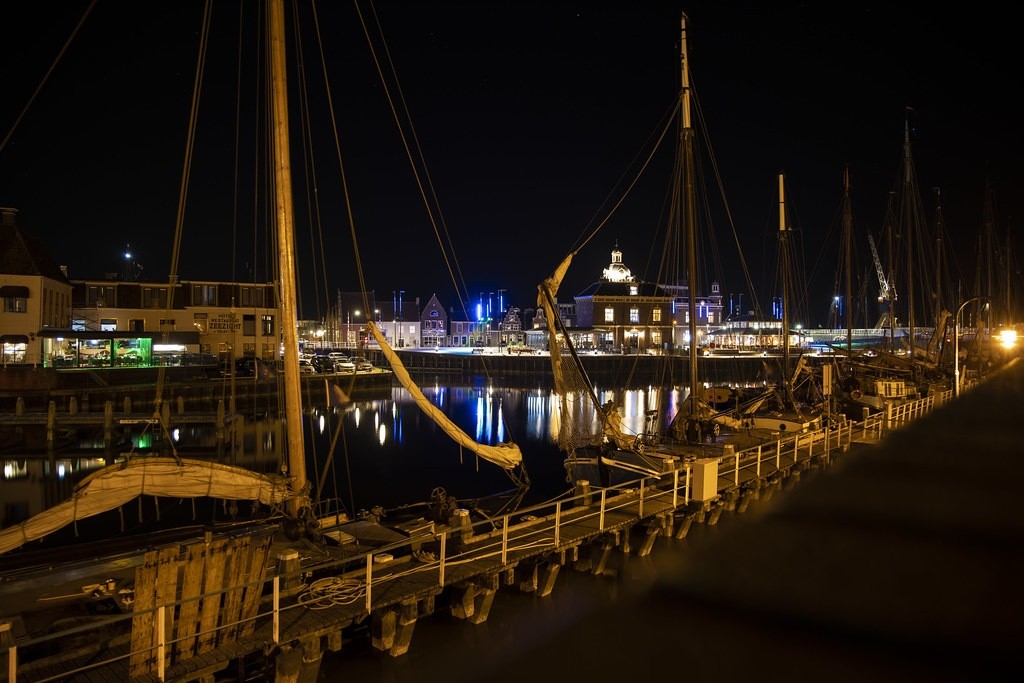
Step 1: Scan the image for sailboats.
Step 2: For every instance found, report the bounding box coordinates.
[531,7,1024,501]
[0,1,534,620]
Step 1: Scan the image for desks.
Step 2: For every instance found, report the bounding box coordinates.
[393,517,436,538]
[82,582,135,613]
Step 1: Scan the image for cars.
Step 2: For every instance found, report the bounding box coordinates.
[219,356,267,378]
[300,360,316,376]
[300,348,373,374]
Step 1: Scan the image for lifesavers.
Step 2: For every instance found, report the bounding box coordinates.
[850,390,863,401]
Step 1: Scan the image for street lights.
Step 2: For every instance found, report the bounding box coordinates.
[497,289,506,353]
[488,292,494,352]
[833,296,840,328]
[738,294,744,347]
[374,309,380,324]
[351,310,361,349]
[955,295,1023,401]
[730,294,736,348]
[479,292,484,347]
[392,291,397,348]
[399,291,405,347]
[796,324,802,348]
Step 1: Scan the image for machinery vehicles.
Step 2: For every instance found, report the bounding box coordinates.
[866,232,899,329]
